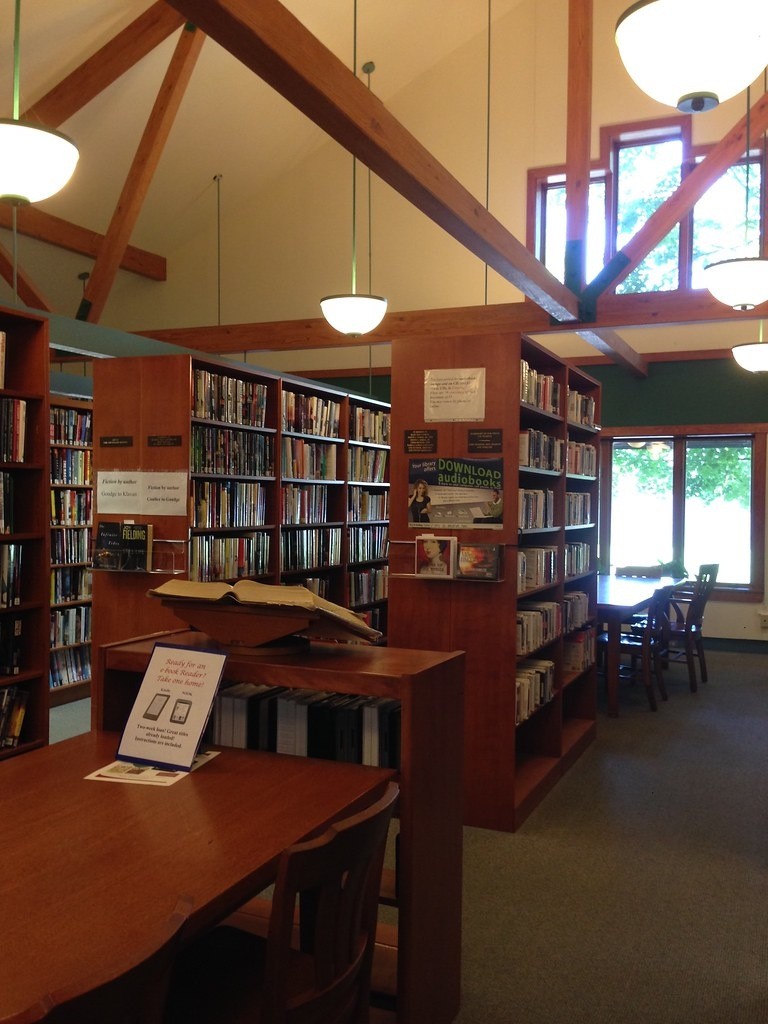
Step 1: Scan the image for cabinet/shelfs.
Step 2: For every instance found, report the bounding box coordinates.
[88,354,392,733]
[389,335,601,833]
[0,307,94,762]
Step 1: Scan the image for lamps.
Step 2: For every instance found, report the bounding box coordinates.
[613,0,768,116]
[731,318,768,373]
[700,86,768,310]
[0,0,80,209]
[319,1,389,336]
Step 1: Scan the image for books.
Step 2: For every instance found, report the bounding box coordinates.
[0,395,27,754]
[189,367,389,646]
[92,521,122,569]
[119,523,153,572]
[145,578,384,642]
[49,404,92,691]
[515,358,600,727]
[413,536,506,579]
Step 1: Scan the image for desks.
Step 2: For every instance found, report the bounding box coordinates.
[594,573,684,717]
[0,729,400,1024]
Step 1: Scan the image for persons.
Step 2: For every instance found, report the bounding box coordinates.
[417,538,450,576]
[406,480,432,524]
[473,488,504,524]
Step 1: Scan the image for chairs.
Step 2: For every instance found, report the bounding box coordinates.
[161,780,403,1024]
[630,564,720,692]
[614,565,660,578]
[594,589,670,712]
